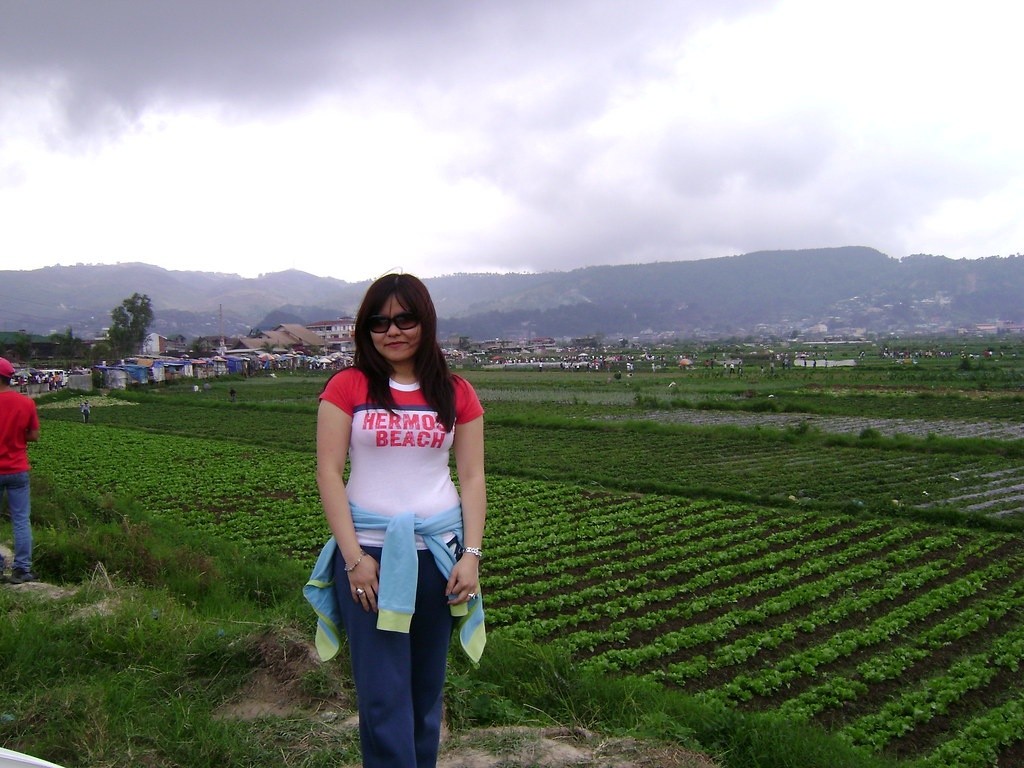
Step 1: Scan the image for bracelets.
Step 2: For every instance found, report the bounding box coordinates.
[344,549,366,572]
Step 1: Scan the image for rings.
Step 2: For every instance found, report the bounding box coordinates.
[468,593,475,599]
[355,588,365,595]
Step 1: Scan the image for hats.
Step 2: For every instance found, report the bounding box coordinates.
[0,357,15,378]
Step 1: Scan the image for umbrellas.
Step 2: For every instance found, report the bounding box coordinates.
[14,368,38,379]
[680,359,693,366]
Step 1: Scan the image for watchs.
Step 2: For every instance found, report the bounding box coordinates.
[460,546,483,558]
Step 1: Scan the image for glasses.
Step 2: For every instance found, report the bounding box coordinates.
[369,312,420,333]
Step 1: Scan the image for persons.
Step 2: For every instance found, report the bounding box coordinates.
[0,357,40,584]
[229,386,237,402]
[302,273,488,768]
[490,350,1016,388]
[10,371,63,392]
[80,400,92,423]
[194,385,199,400]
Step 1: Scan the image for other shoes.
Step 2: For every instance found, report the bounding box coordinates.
[0,571,9,583]
[9,569,25,583]
